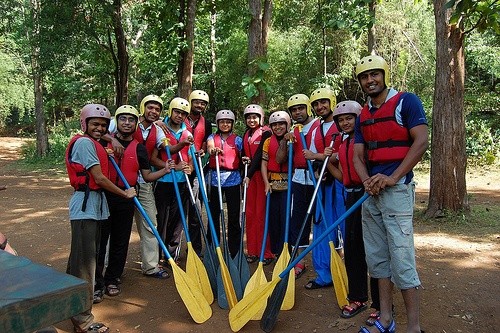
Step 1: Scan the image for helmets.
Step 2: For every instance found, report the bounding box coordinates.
[168,97,190,121]
[310,88,336,117]
[244,104,264,126]
[115,105,139,131]
[216,110,235,132]
[269,111,291,132]
[356,55,390,92]
[189,90,209,113]
[333,100,363,132]
[140,95,163,116]
[80,104,111,132]
[287,93,312,120]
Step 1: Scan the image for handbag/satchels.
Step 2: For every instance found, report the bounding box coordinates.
[309,161,335,185]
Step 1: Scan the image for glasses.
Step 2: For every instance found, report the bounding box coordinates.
[291,106,305,112]
[118,116,135,122]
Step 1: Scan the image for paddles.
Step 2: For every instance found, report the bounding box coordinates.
[106,124,371,332]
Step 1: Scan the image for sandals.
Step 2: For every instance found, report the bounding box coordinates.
[263,258,273,266]
[247,255,258,263]
[85,323,110,333]
[143,270,171,278]
[304,280,333,289]
[358,319,396,333]
[294,263,307,278]
[105,284,121,296]
[340,298,368,318]
[366,309,395,326]
[93,289,104,302]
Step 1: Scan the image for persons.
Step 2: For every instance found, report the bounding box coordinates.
[153,97,205,269]
[242,104,275,266]
[302,88,345,289]
[261,111,294,261]
[275,94,324,278]
[352,55,429,333]
[93,105,175,303]
[206,109,245,259]
[155,89,212,257]
[66,104,137,333]
[101,94,194,284]
[324,100,395,325]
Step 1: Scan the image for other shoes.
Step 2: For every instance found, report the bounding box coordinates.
[164,261,172,269]
[198,254,205,263]
